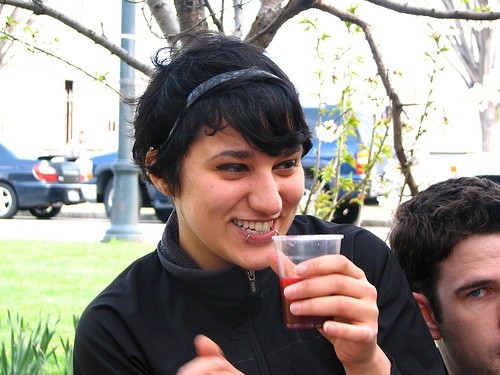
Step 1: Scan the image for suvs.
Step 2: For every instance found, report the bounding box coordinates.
[80,104,372,224]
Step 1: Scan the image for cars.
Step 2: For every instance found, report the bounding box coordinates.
[0,136,87,218]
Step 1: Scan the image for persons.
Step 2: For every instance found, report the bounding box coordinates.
[72,37,448,374]
[389,176,500,375]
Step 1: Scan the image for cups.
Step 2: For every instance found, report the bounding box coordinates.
[271,234,345,331]
